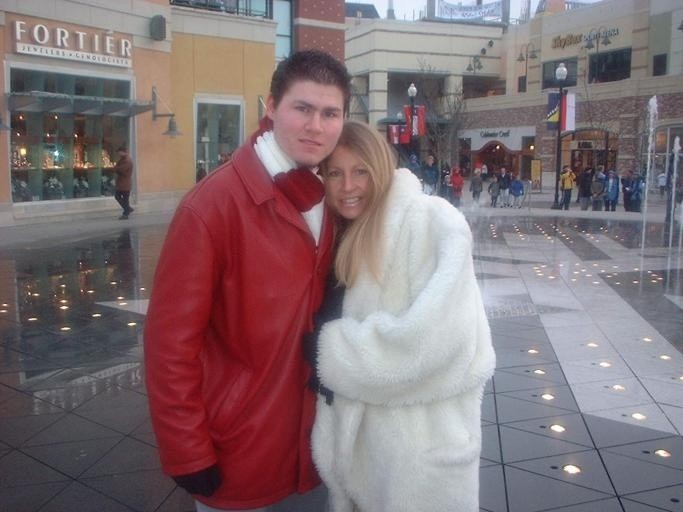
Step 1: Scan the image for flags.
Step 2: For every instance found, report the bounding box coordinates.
[403,102,426,137]
[388,125,411,145]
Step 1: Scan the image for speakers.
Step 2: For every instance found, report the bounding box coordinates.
[151,15,166,41]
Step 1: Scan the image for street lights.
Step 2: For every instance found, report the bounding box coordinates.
[396,111,404,167]
[515,41,539,91]
[549,60,569,211]
[583,25,613,84]
[406,80,419,154]
[465,54,483,98]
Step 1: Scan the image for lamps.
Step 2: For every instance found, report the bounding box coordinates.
[481,40,494,55]
[151,85,186,139]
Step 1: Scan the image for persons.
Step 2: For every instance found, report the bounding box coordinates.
[402,152,525,210]
[559,164,645,211]
[301,119,497,510]
[143,48,348,510]
[112,147,134,220]
[658,171,666,196]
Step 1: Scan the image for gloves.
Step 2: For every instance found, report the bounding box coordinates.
[300,311,327,372]
[173,463,220,497]
